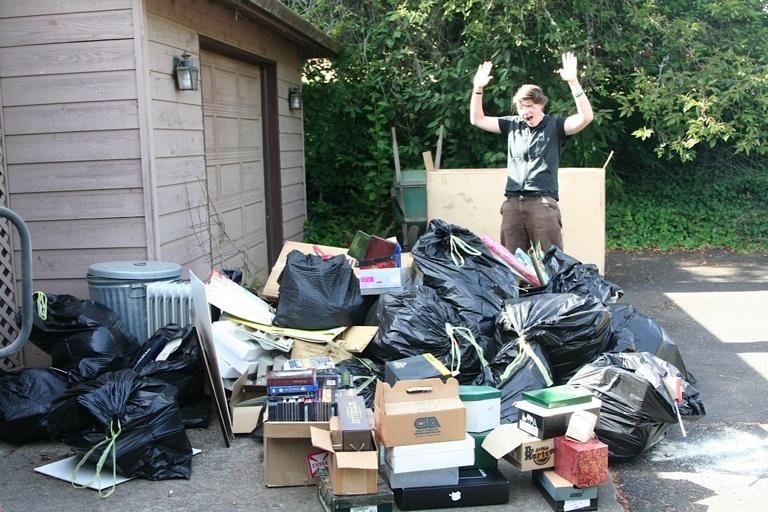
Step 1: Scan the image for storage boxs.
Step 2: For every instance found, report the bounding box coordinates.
[229,354,610,511]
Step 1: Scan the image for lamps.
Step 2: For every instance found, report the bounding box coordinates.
[176,52,199,93]
[287,86,305,108]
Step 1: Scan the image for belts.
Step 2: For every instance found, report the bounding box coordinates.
[505,191,559,200]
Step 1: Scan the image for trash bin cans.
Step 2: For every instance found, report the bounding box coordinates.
[87,260,182,345]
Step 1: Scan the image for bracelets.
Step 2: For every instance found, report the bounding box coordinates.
[472,90,484,95]
[573,88,584,99]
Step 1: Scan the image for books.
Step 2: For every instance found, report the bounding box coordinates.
[266,369,332,421]
[357,266,403,295]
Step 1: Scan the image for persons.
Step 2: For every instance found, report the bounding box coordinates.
[469,51,594,256]
